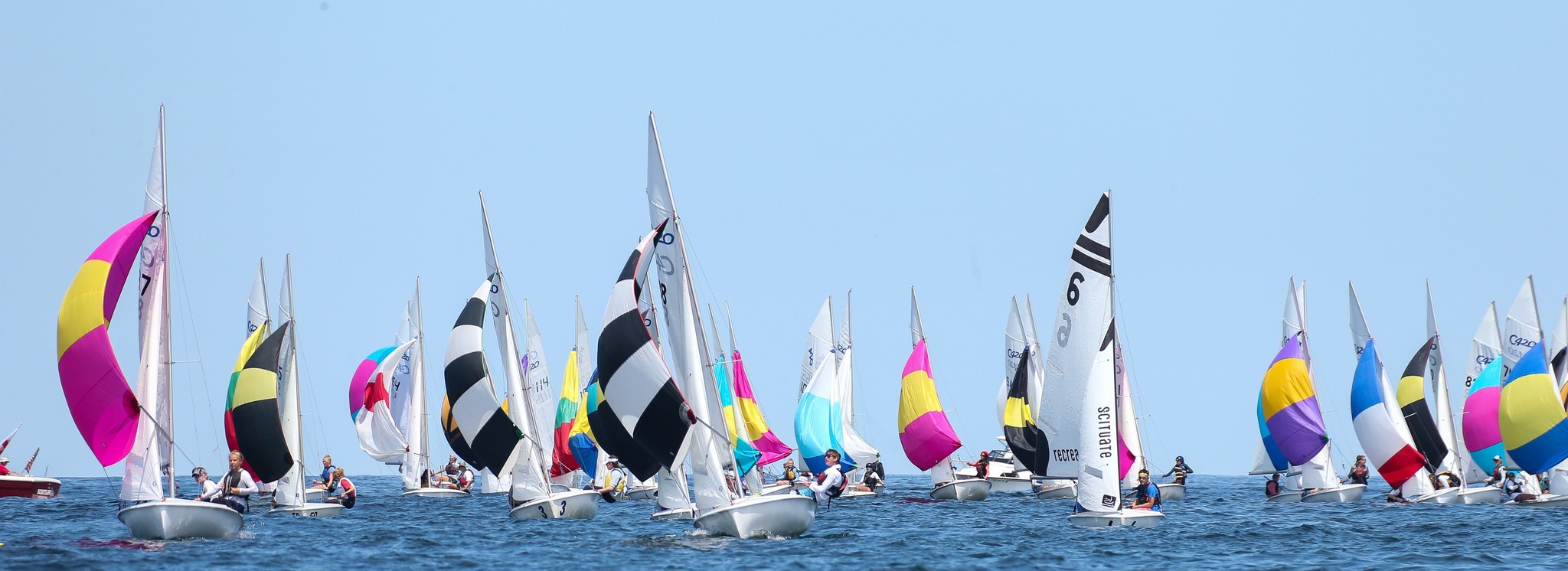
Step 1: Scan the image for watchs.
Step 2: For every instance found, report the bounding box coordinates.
[343,492,346,495]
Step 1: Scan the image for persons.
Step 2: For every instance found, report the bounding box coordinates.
[1265,474,1281,498]
[420,469,442,488]
[855,463,882,492]
[447,461,474,494]
[310,455,337,493]
[192,465,223,502]
[326,467,356,509]
[1505,472,1536,501]
[872,459,885,484]
[1483,455,1508,489]
[433,454,460,484]
[1340,455,1370,486]
[0,457,20,475]
[272,488,280,506]
[1122,469,1161,512]
[1387,485,1407,502]
[194,450,259,514]
[1537,472,1550,494]
[790,449,848,505]
[1162,456,1193,486]
[968,451,990,479]
[583,456,627,503]
[777,458,800,486]
[1429,471,1461,490]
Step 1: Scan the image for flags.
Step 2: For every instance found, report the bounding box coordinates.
[25,448,39,473]
[0,428,18,455]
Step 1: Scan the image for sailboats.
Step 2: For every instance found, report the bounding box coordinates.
[1248,276,1368,505]
[57,104,244,540]
[1349,274,1568,505]
[897,188,1185,527]
[223,255,344,519]
[348,110,884,541]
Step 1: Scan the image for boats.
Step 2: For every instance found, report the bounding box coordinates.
[0,475,62,499]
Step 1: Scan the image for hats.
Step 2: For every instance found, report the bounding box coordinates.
[0,457,10,463]
[192,469,206,478]
[458,461,467,469]
[602,458,619,465]
[450,454,458,459]
[1493,455,1502,460]
[981,451,989,456]
[1272,474,1281,478]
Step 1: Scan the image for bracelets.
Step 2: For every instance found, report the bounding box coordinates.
[200,496,203,500]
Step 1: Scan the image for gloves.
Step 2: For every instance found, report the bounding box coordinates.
[790,482,795,486]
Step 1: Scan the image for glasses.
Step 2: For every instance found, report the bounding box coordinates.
[980,454,985,456]
[1138,477,1146,479]
[866,467,872,468]
[1275,477,1280,478]
[1508,475,1514,478]
[824,454,836,459]
[1494,460,1500,462]
[1362,458,1366,461]
[449,458,453,459]
[783,466,787,468]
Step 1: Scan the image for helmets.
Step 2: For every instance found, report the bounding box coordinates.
[866,463,873,468]
[1176,456,1184,461]
